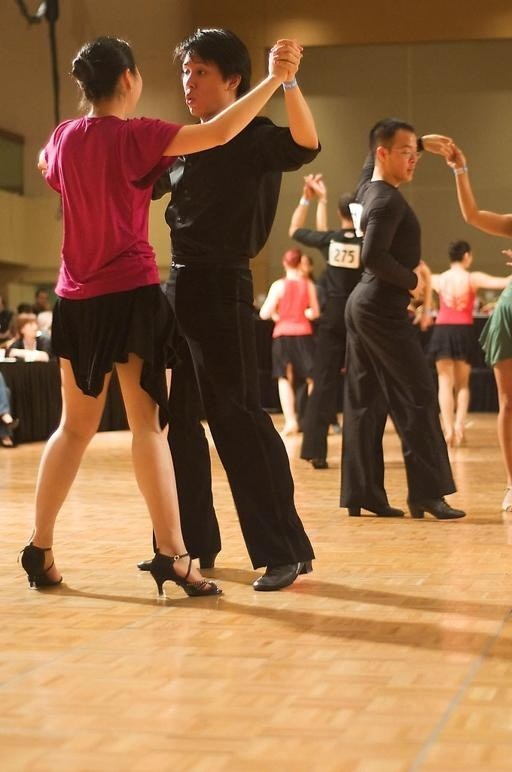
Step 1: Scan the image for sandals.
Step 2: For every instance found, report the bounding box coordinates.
[278,424,300,438]
[501,486,512,513]
[444,427,466,448]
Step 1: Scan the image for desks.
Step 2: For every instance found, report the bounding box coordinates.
[1,353,175,446]
[254,313,499,416]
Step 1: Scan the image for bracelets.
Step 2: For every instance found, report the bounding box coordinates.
[282,78,297,89]
[453,167,468,175]
[422,308,433,316]
[299,197,311,206]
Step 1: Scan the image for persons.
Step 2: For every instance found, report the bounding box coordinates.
[440,144,512,512]
[259,248,322,436]
[430,239,512,447]
[288,172,365,471]
[0,289,54,448]
[304,177,432,331]
[339,118,465,520]
[271,253,341,434]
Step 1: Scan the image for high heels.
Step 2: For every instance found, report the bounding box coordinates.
[21,542,63,588]
[407,495,469,519]
[252,559,314,592]
[149,548,223,597]
[0,412,21,448]
[348,501,404,516]
[137,551,218,570]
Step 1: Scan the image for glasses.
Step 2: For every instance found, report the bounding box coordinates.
[385,144,423,160]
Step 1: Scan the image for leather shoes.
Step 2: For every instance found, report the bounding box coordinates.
[301,455,329,469]
